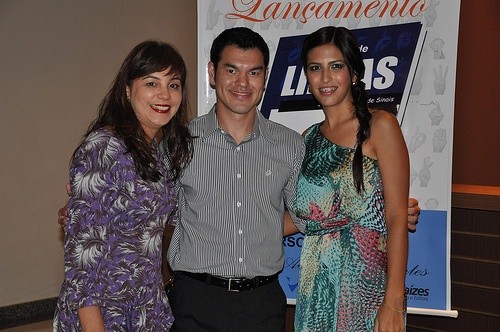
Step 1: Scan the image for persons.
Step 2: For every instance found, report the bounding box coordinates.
[284,26,410,332]
[53,41,195,332]
[65,27,420,332]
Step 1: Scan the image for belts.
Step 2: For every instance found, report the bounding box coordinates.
[179,271,278,292]
